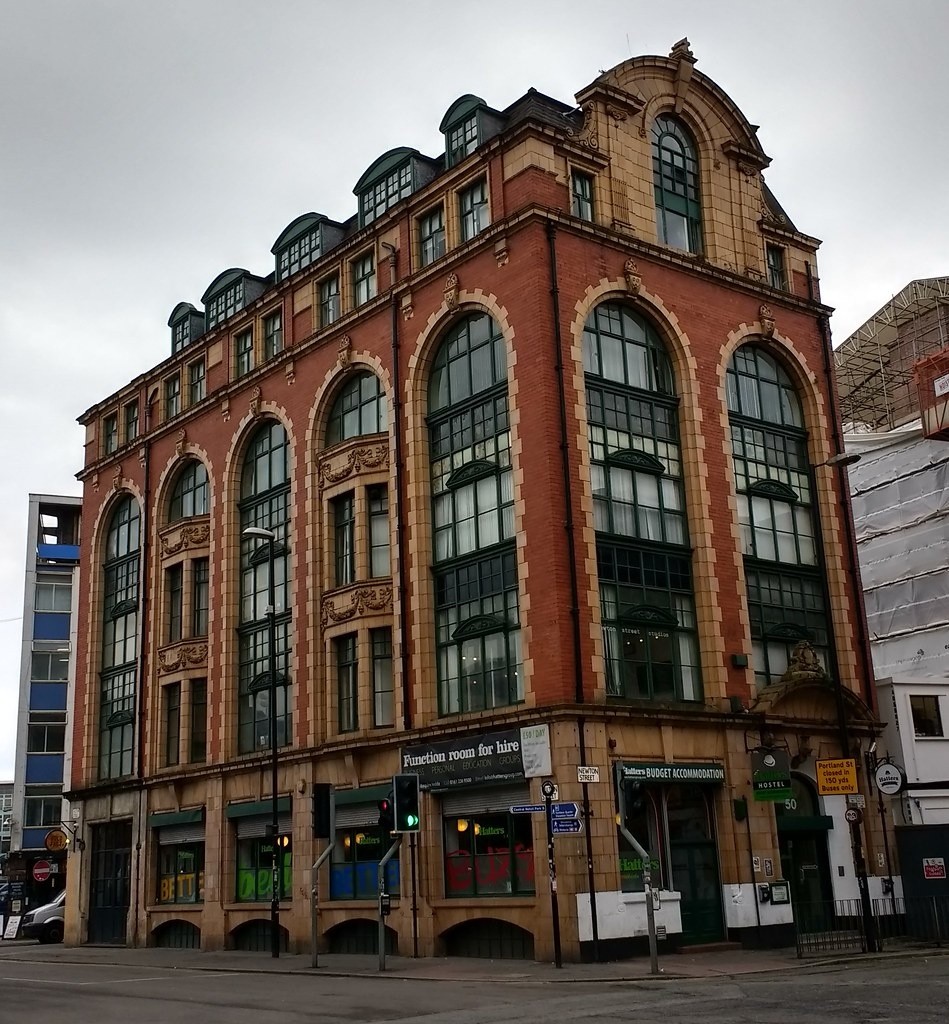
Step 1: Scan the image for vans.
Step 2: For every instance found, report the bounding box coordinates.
[0,882,10,909]
[21,888,67,944]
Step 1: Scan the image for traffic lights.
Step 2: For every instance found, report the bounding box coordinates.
[377,796,394,834]
[393,772,420,833]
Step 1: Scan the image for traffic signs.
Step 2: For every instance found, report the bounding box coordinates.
[551,802,580,819]
[509,804,547,814]
[550,817,584,835]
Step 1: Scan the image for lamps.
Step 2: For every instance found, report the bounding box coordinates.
[4,817,11,825]
[868,741,878,753]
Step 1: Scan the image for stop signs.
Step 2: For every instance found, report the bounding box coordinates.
[33,859,52,881]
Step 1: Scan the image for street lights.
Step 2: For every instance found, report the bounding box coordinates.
[813,446,880,955]
[245,526,281,958]
